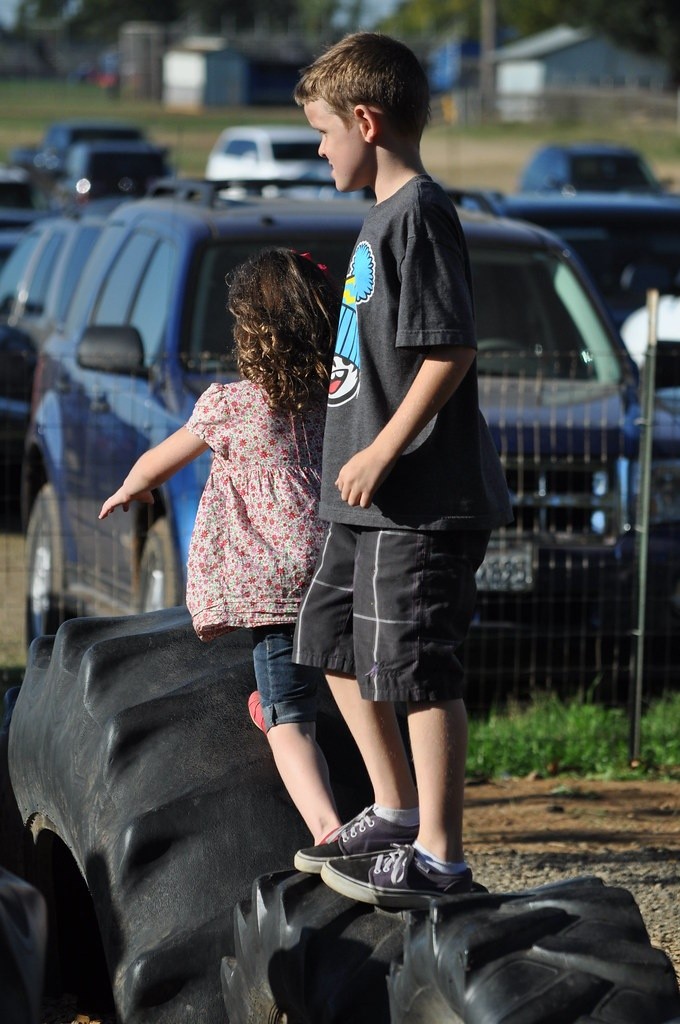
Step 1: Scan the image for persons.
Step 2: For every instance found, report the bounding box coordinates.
[97,246,343,848]
[293,32,515,908]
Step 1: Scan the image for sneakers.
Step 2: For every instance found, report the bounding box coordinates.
[293,804,420,874]
[320,842,472,908]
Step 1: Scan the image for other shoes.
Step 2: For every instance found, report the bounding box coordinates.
[248,690,269,733]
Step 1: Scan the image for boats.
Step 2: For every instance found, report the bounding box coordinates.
[0,121,171,227]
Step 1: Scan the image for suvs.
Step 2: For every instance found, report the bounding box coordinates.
[446,186,678,550]
[18,178,680,710]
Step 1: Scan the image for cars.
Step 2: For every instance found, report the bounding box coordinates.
[516,144,669,194]
[202,126,375,202]
[0,200,127,449]
